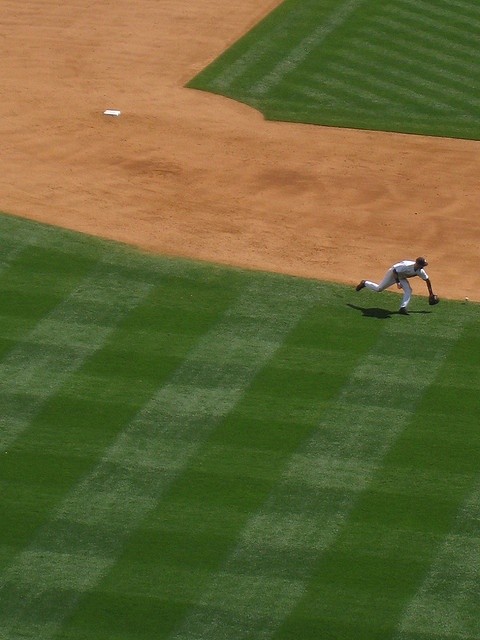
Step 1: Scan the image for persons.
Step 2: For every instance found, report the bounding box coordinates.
[355,257,438,315]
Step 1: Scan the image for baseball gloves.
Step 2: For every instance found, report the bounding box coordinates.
[429,294,439,305]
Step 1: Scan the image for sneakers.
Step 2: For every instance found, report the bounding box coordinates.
[356,280,366,291]
[399,307,409,315]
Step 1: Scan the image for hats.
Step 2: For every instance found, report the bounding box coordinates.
[416,257,428,266]
